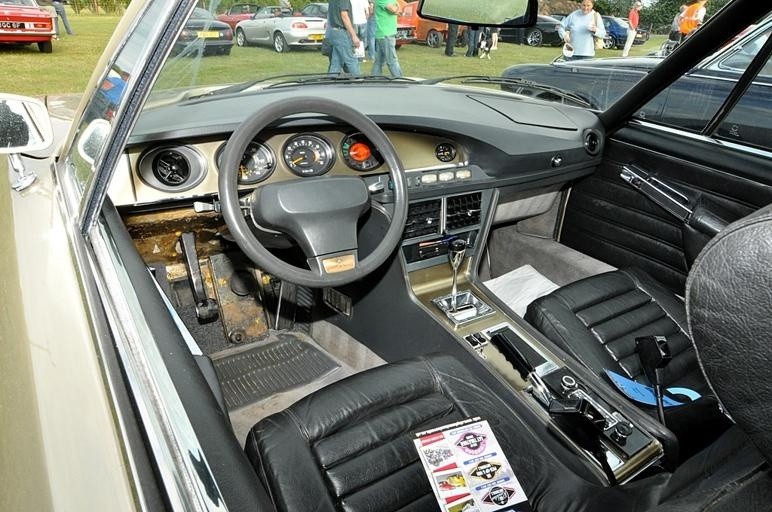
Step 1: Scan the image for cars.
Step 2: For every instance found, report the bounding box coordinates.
[0,2,771,509]
[502,13,771,152]
[1,1,57,54]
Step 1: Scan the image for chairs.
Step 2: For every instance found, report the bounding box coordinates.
[244,204,772,511]
[524,265,733,465]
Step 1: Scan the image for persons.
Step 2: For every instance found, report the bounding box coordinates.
[678,0,708,44]
[86,71,130,122]
[444,24,501,57]
[321,0,403,79]
[669,5,688,44]
[558,0,606,61]
[52,0,76,36]
[622,1,641,57]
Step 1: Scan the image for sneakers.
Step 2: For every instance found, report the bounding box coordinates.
[480,52,486,58]
